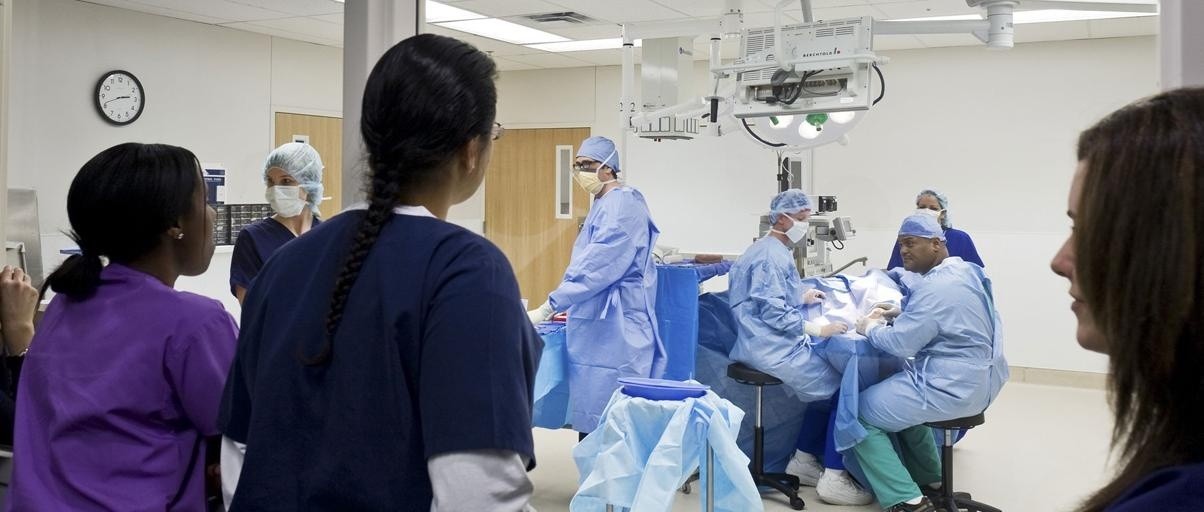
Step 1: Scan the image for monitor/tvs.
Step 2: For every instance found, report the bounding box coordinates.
[733,17,876,116]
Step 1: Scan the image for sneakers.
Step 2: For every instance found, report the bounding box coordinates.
[816,470,873,506]
[785,457,823,486]
[890,485,943,511]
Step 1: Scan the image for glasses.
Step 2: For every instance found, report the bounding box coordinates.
[490,121,503,141]
[574,159,598,170]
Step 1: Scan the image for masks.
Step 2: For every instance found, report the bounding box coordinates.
[780,214,810,244]
[265,187,311,217]
[916,207,945,219]
[571,163,608,194]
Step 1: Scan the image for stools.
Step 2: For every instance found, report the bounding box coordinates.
[923,416,1003,512]
[726,361,807,511]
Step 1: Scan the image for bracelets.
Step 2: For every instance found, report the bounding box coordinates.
[6,348,29,356]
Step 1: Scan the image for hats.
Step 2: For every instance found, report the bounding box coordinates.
[898,213,946,242]
[917,188,948,207]
[769,189,811,227]
[577,135,619,172]
[262,141,324,206]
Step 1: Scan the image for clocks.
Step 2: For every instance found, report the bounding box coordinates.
[94,69,146,127]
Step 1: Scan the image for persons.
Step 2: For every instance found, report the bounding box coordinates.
[6,143,241,511]
[886,188,985,442]
[521,134,667,439]
[230,142,327,305]
[729,188,873,508]
[218,32,544,510]
[1,266,39,512]
[852,214,1009,512]
[1049,84,1204,511]
